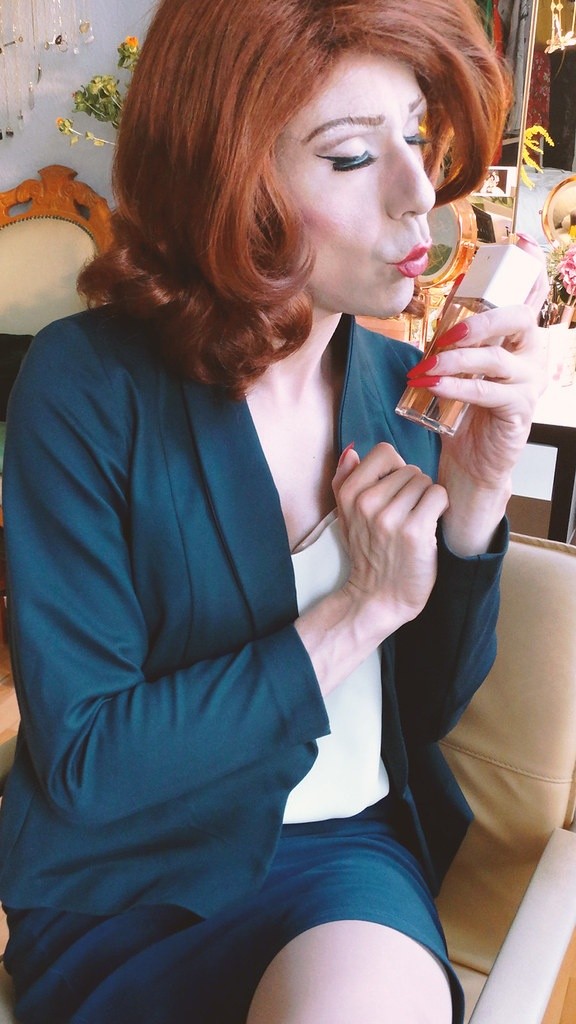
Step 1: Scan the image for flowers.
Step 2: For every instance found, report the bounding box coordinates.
[55,33,145,148]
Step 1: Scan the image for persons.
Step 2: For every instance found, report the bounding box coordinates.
[0,0,551,1024]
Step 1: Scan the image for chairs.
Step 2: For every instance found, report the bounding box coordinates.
[0,164,115,338]
[0,531,576,1024]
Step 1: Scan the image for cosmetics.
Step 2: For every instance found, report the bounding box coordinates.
[396,243,543,437]
